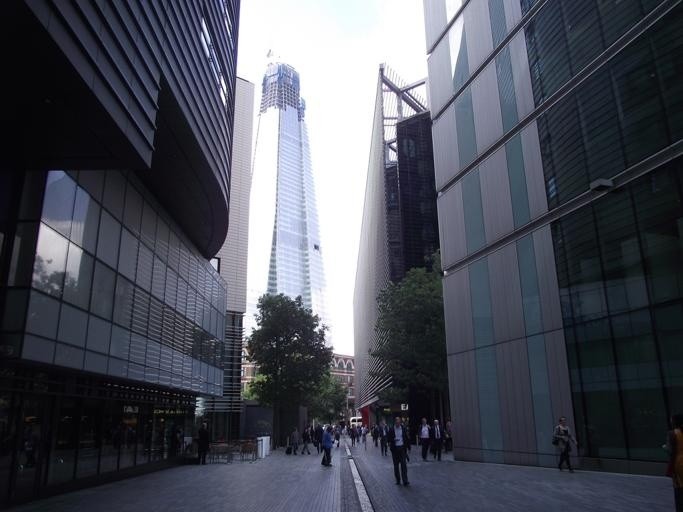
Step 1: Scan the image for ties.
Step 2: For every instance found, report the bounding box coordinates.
[436,426,439,438]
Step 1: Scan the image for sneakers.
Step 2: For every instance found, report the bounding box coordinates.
[321,462,333,467]
[396,481,410,487]
[294,451,311,456]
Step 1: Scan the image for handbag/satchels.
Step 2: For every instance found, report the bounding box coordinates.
[552,426,560,446]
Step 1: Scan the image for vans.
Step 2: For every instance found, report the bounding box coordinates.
[350,417,362,429]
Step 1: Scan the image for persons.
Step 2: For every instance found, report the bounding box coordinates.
[387,416,413,486]
[198,422,209,465]
[286,417,452,468]
[656,413,683,512]
[552,416,578,473]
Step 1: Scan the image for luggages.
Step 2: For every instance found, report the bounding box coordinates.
[285,444,293,455]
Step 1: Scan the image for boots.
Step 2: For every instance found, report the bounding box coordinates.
[566,461,575,474]
[558,458,564,472]
